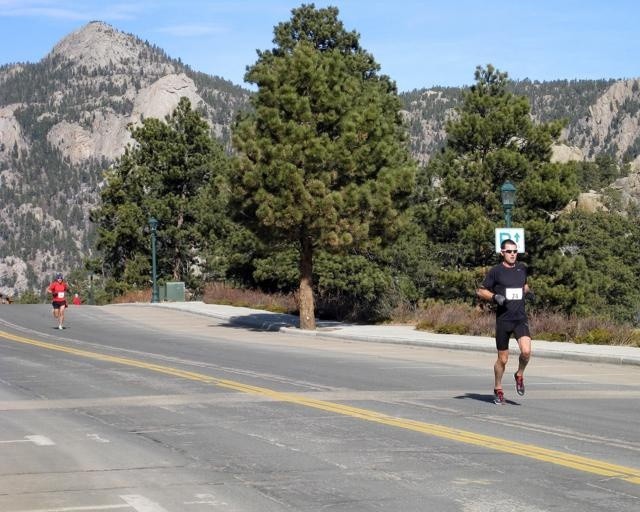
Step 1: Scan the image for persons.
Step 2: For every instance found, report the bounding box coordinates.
[474,239,533,405]
[46,273,72,329]
[73,296,80,306]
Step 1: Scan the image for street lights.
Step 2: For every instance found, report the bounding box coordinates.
[148,215,160,302]
[500,179,517,227]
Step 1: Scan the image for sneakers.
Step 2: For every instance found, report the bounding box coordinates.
[493,388,504,404]
[514,372,524,396]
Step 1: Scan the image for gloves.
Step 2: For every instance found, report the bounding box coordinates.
[492,294,506,306]
[524,291,536,302]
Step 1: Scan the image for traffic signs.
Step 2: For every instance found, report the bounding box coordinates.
[495,227,525,254]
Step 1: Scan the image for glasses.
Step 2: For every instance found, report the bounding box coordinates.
[501,249,517,253]
